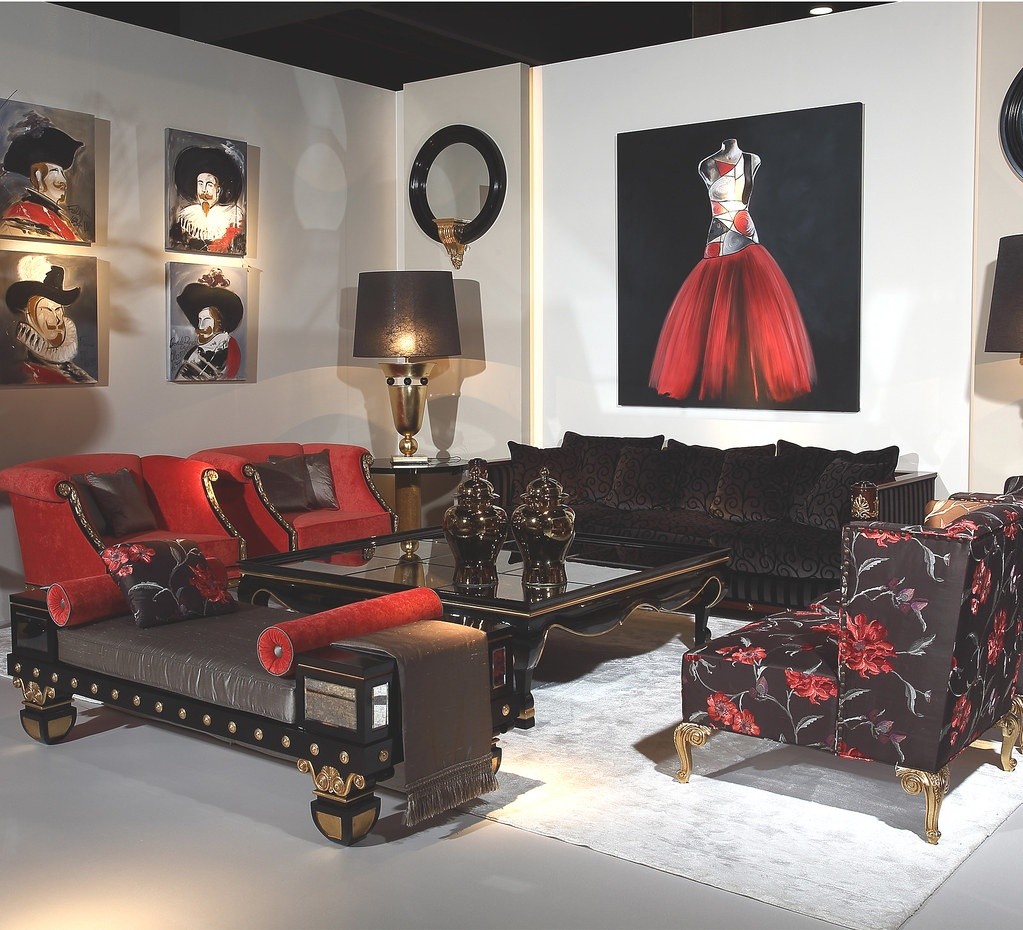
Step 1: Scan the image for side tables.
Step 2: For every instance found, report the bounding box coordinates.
[369,457,468,533]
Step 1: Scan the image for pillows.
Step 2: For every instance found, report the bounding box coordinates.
[250,454,320,511]
[70,470,112,536]
[83,466,155,539]
[709,453,800,523]
[97,538,240,628]
[596,445,689,511]
[508,440,592,504]
[666,439,776,513]
[265,448,340,511]
[257,587,444,676]
[561,431,665,504]
[46,556,229,628]
[777,440,900,531]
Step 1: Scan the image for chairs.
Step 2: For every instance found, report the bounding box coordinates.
[189,443,399,559]
[0,453,247,593]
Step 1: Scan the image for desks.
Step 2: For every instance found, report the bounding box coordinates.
[235,524,731,730]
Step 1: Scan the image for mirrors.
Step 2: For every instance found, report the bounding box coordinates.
[408,124,508,271]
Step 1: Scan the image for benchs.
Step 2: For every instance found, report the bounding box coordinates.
[674,476,1023,846]
[470,457,938,616]
[6,588,503,846]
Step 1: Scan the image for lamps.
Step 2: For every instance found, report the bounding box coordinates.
[351,271,461,457]
[985,234,1023,366]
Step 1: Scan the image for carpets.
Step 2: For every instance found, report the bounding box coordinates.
[1,609,1023,929]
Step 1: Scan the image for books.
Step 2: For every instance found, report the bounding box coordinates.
[392,455,430,468]
